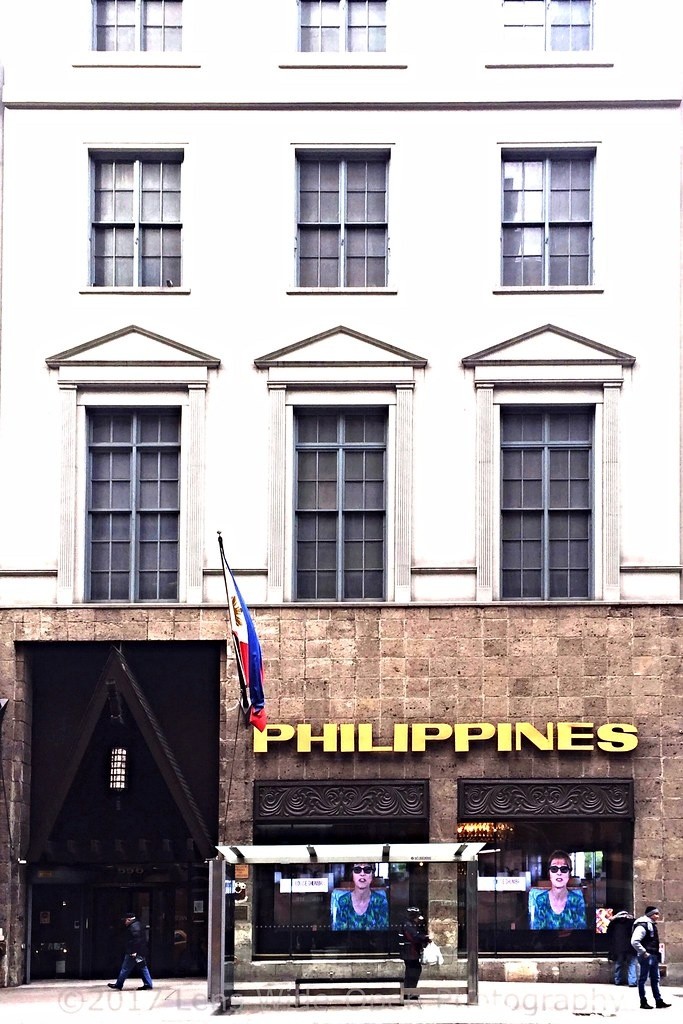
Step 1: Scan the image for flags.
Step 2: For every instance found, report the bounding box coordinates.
[220,548,269,732]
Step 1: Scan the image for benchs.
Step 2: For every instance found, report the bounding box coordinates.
[295,977,405,1007]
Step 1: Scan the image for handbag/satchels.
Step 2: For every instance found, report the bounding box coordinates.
[422,942,444,966]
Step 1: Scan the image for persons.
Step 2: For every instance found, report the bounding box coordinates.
[608,907,639,987]
[108,914,153,991]
[399,908,432,1000]
[331,864,388,932]
[631,906,672,1009]
[532,850,588,930]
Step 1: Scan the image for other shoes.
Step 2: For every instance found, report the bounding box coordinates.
[137,985,152,990]
[641,999,653,1009]
[404,994,418,1000]
[656,999,671,1007]
[108,983,121,990]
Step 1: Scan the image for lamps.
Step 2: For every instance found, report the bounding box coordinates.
[111,738,127,812]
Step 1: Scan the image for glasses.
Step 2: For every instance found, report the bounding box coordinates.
[654,913,658,915]
[548,865,572,873]
[351,866,373,875]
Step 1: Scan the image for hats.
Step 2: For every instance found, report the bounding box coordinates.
[121,912,135,920]
[645,906,658,916]
